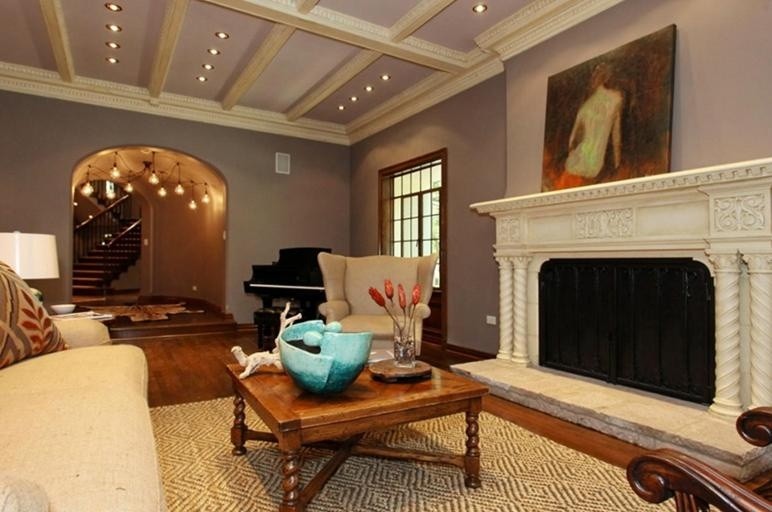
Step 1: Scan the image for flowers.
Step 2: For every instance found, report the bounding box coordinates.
[368,279,421,320]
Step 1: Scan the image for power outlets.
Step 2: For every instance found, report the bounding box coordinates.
[485,316,496,326]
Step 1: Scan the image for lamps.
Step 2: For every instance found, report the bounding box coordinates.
[0,232,60,281]
[188,200,197,210]
[149,170,160,185]
[107,186,116,199]
[124,180,134,193]
[80,182,94,197]
[202,190,210,204]
[174,183,184,195]
[158,185,167,197]
[110,164,120,179]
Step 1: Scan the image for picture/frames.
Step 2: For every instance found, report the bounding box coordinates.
[540,24,677,193]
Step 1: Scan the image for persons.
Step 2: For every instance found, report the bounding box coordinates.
[565,59,624,181]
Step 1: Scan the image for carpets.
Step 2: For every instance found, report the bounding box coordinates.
[149,396,716,512]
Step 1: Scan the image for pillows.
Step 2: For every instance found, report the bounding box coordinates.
[0,261,71,370]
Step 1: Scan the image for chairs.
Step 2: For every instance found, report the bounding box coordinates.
[626,405,772,512]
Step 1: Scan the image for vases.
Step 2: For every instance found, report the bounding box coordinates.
[393,321,416,368]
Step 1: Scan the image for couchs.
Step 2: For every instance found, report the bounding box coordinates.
[318,252,438,356]
[0,317,168,512]
